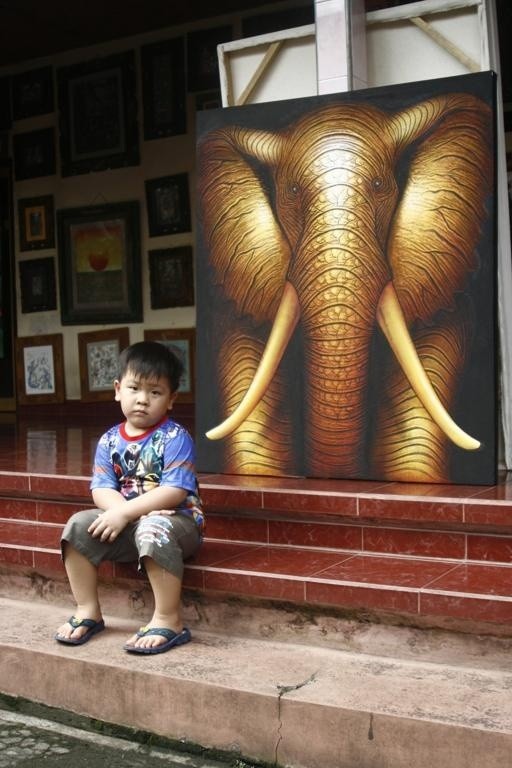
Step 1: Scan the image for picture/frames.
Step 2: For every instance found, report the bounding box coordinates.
[2,0,271,62]
[57,200,145,325]
[13,126,56,182]
[240,4,315,38]
[139,36,188,141]
[143,328,197,406]
[78,328,129,404]
[16,194,55,252]
[195,91,223,113]
[60,49,141,178]
[9,65,55,120]
[144,172,192,239]
[19,258,57,315]
[185,24,232,92]
[16,333,67,406]
[150,246,194,310]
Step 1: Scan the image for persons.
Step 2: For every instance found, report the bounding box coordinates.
[54,341,206,654]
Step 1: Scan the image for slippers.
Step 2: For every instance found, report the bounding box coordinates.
[122,623,192,653]
[52,616,106,646]
